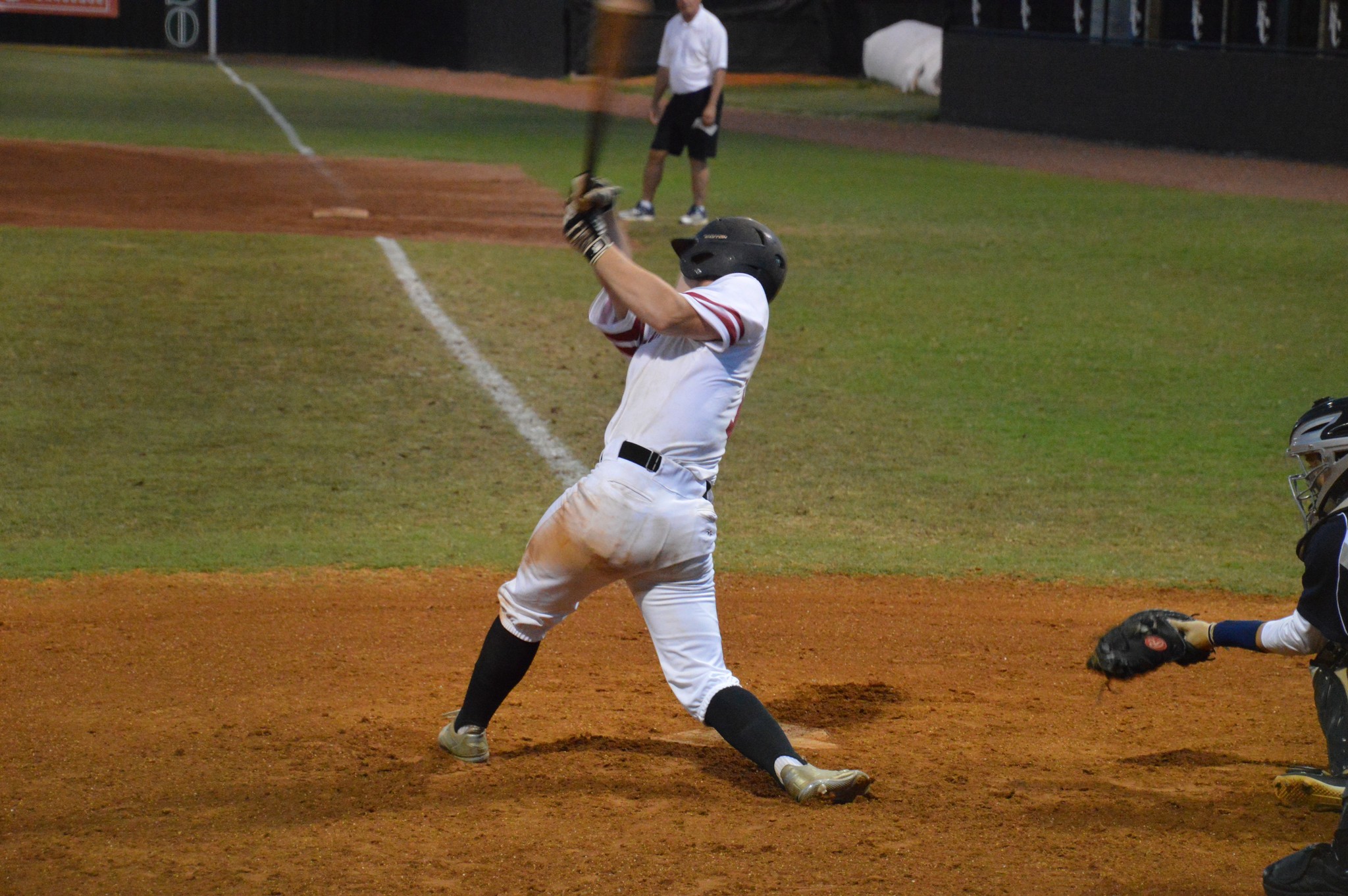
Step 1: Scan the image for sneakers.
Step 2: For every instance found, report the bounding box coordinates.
[1272,764,1348,811]
[780,760,872,804]
[437,710,489,762]
[679,204,708,226]
[617,202,658,221]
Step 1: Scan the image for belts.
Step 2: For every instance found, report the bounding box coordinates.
[618,440,712,499]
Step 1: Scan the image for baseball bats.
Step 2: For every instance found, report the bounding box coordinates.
[581,2,649,198]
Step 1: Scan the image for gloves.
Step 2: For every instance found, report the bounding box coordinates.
[560,201,614,267]
[573,172,621,217]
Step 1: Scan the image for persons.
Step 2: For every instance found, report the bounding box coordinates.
[437,176,876,807]
[616,0,729,225]
[1083,395,1348,815]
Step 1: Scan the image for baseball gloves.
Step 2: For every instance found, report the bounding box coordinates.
[1086,610,1217,682]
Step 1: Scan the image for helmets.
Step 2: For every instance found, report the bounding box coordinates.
[1286,397,1348,530]
[672,217,788,304]
